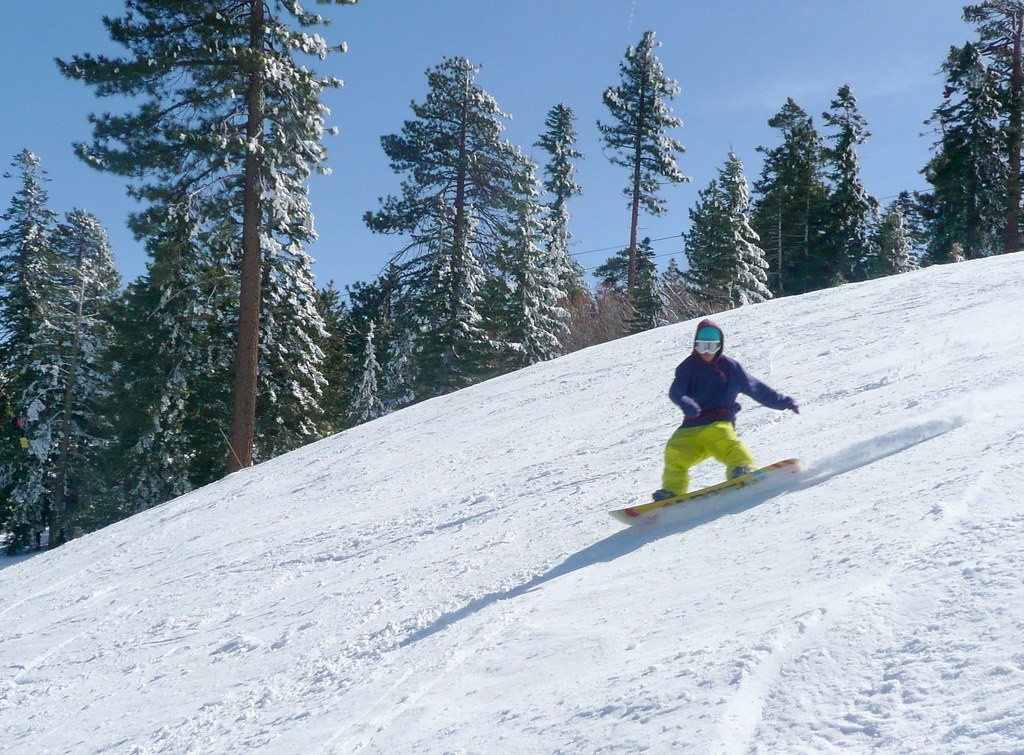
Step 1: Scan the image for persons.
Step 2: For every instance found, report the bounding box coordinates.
[654,318,800,502]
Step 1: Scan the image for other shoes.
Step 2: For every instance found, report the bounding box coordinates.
[653,489,675,502]
[732,467,749,478]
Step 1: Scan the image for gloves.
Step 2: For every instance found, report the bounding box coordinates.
[682,399,702,419]
[778,396,800,414]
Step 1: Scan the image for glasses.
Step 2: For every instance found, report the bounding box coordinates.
[695,341,721,355]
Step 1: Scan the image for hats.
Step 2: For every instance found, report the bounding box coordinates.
[697,327,721,341]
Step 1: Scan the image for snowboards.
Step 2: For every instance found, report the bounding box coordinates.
[607,456,807,524]
